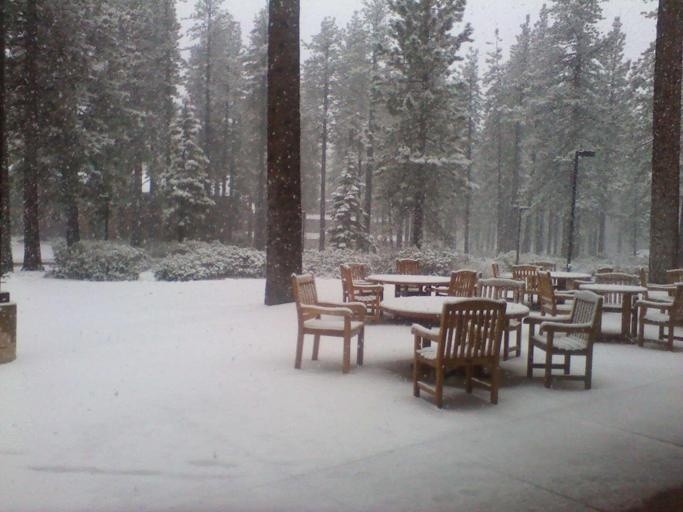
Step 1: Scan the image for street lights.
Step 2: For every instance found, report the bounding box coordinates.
[566,150,596,272]
